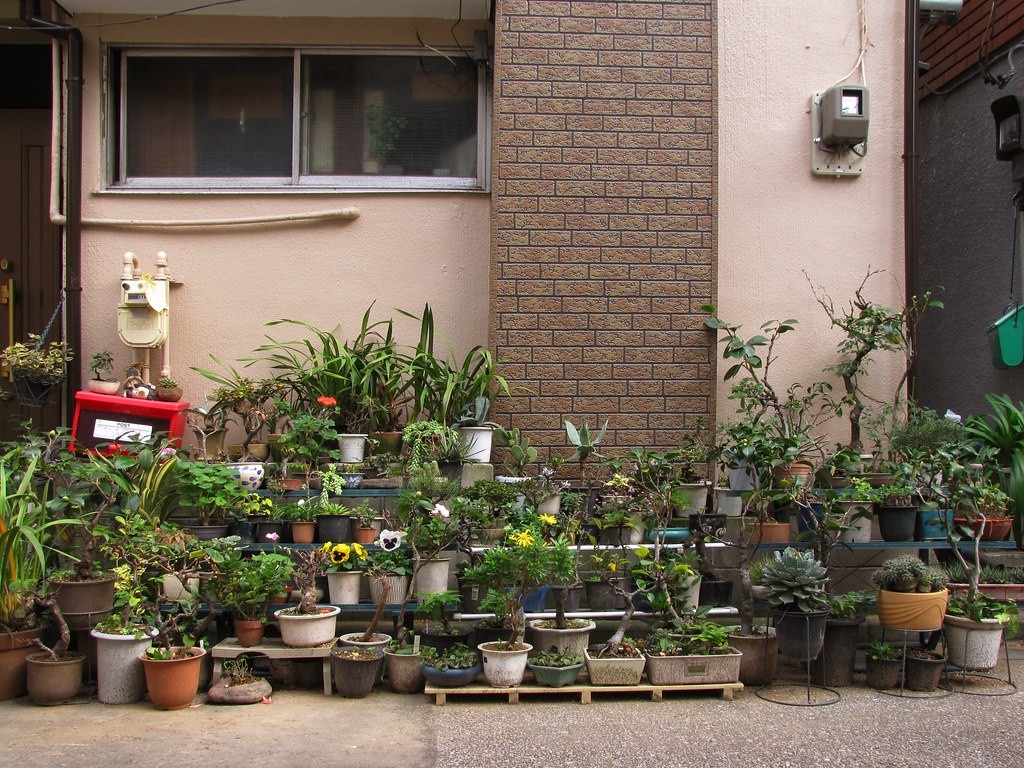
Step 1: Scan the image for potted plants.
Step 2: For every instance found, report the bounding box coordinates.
[0,264,1024,710]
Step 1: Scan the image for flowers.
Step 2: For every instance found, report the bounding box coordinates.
[502,509,559,586]
[363,528,415,575]
[252,533,298,599]
[316,541,370,572]
[271,396,342,520]
[595,474,640,496]
[592,486,654,533]
[407,504,460,559]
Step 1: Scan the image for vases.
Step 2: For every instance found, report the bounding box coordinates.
[630,513,646,545]
[515,587,550,612]
[291,522,316,544]
[598,529,629,546]
[367,576,410,604]
[327,570,363,605]
[412,557,452,605]
[268,585,294,605]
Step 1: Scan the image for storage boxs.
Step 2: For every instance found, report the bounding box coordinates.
[68,391,190,463]
